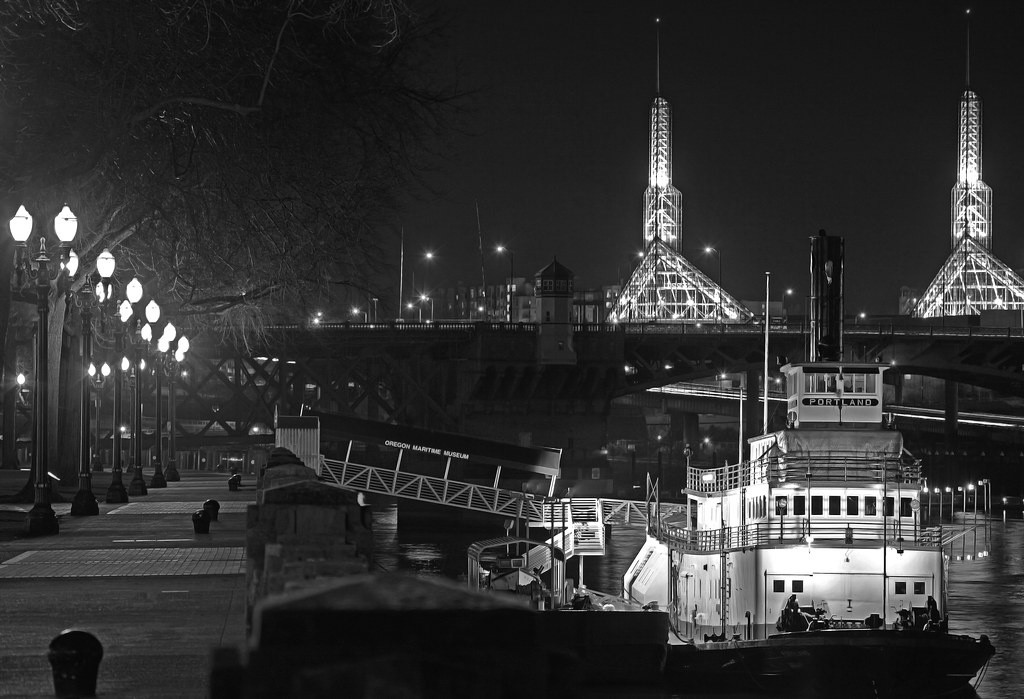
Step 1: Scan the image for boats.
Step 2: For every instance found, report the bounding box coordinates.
[622,273,999,699]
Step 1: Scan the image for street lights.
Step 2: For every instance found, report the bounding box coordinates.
[9,202,80,537]
[58,247,117,515]
[703,436,717,468]
[977,480,987,513]
[657,435,663,465]
[780,290,793,327]
[706,247,723,325]
[957,486,965,522]
[855,313,866,326]
[353,309,368,324]
[966,529,977,561]
[946,486,954,525]
[968,484,978,520]
[372,298,378,323]
[419,295,434,323]
[923,487,931,526]
[408,303,422,325]
[94,276,190,503]
[983,478,992,514]
[498,246,513,332]
[934,488,943,525]
[421,253,433,293]
[982,518,988,558]
[956,535,966,562]
